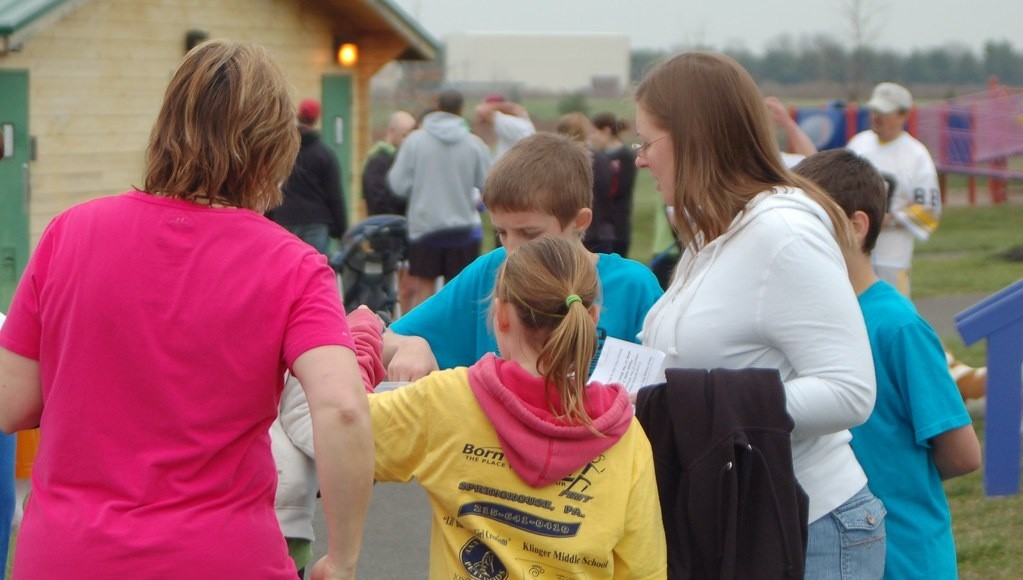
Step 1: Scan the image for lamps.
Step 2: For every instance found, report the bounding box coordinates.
[335,34,359,67]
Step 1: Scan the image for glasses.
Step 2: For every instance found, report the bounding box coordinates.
[631,131,672,157]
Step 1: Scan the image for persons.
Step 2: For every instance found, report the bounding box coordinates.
[763,96,819,169]
[558,111,635,257]
[269,371,320,580]
[0,39,375,580]
[381,132,664,383]
[363,91,535,317]
[268,99,346,251]
[792,150,981,580]
[622,49,886,580]
[347,238,667,580]
[847,82,943,295]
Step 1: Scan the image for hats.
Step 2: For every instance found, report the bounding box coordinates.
[299,97,321,122]
[865,81,913,115]
